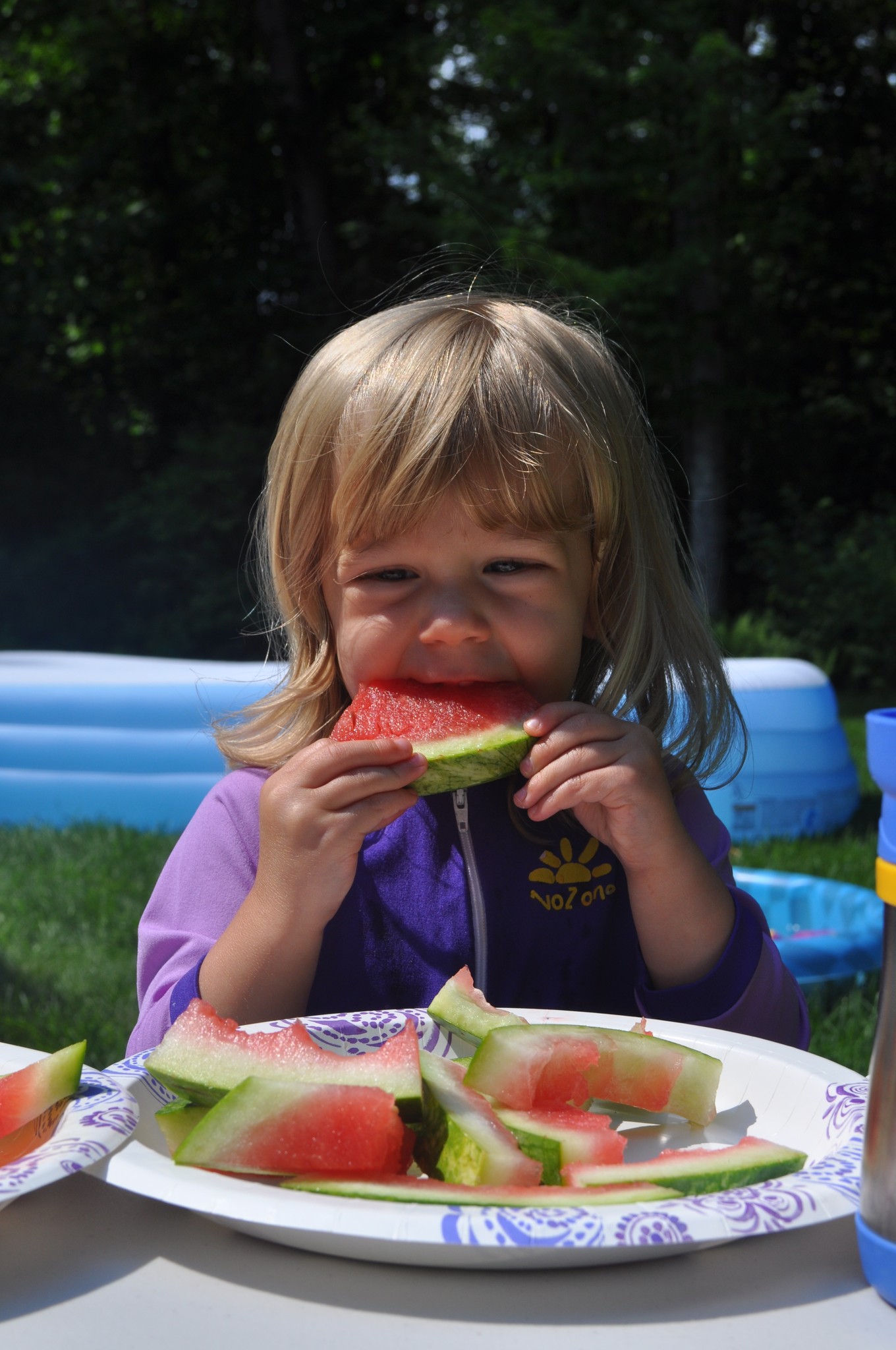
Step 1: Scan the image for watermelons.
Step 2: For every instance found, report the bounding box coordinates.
[328,679,546,794]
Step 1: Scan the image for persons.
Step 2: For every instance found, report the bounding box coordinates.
[127,289,811,1060]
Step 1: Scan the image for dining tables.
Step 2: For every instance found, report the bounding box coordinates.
[0,1172,896,1350]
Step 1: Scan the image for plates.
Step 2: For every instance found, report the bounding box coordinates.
[1,1043,142,1212]
[80,1007,869,1268]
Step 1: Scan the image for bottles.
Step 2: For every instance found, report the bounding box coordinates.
[854,707,896,1307]
